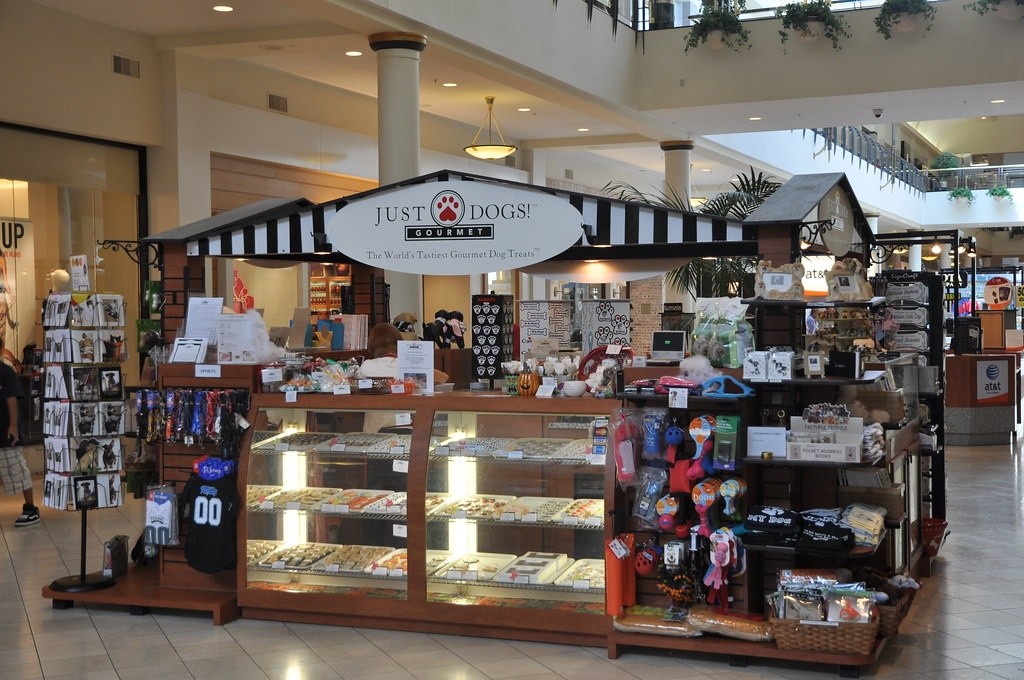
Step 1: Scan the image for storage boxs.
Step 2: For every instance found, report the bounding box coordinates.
[786,415,864,463]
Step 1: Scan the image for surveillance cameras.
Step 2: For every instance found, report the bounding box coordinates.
[873,109,884,118]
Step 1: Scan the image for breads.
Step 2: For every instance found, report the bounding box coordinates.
[616,604,774,636]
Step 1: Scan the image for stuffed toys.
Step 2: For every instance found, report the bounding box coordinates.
[393,310,467,349]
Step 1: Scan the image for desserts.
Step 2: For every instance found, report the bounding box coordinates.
[246,431,606,589]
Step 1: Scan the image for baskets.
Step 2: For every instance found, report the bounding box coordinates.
[879,593,910,638]
[768,603,879,654]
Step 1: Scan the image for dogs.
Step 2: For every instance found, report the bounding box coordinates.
[393,311,420,341]
[422,310,465,349]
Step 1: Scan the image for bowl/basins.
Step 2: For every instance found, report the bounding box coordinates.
[564,380,587,397]
[388,381,414,395]
[350,379,388,394]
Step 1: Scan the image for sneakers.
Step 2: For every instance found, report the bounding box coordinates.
[15,503,41,525]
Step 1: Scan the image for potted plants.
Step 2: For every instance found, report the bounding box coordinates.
[682,6,753,57]
[946,186,977,209]
[985,184,1016,208]
[961,0,1024,23]
[773,0,854,56]
[872,0,938,41]
[929,151,961,178]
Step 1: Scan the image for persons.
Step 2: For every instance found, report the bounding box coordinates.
[0,337,40,527]
[359,323,449,546]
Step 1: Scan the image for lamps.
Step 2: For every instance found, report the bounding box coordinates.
[462,96,519,161]
[967,243,977,257]
[958,245,966,254]
[947,246,955,259]
[931,241,941,254]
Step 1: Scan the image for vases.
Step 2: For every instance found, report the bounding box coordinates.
[939,182,948,187]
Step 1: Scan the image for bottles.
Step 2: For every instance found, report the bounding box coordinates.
[304,313,344,351]
[308,263,351,310]
[140,357,152,387]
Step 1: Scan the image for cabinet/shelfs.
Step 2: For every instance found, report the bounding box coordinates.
[815,317,870,340]
[875,438,924,581]
[236,392,624,648]
[617,394,889,557]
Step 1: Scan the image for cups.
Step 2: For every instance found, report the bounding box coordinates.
[517,374,538,397]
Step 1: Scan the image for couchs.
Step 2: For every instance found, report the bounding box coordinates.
[1003,172,1024,188]
[964,172,998,190]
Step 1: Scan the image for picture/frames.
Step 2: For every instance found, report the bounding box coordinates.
[823,257,874,302]
[754,259,806,300]
[802,350,825,376]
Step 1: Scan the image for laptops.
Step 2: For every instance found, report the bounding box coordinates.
[646,330,686,364]
